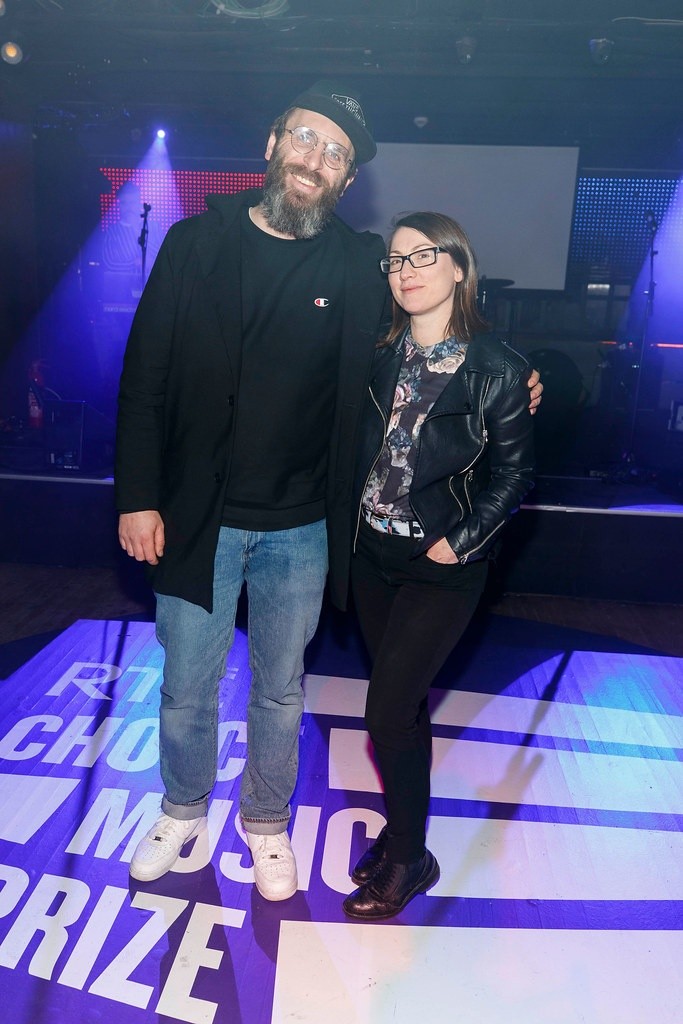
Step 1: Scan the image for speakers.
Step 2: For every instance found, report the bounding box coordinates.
[41,398,117,470]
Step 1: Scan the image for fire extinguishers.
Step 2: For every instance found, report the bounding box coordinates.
[26,358,51,429]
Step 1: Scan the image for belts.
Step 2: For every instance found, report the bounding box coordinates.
[361,508,424,538]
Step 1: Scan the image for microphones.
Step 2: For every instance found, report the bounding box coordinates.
[645,209,658,231]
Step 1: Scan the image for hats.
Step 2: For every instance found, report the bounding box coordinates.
[290,79,377,165]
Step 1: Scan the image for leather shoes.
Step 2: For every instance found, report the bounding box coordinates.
[343,848,440,919]
[352,823,394,886]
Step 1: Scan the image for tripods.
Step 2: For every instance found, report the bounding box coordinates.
[606,229,668,486]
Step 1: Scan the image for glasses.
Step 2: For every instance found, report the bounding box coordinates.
[379,247,448,274]
[281,126,353,171]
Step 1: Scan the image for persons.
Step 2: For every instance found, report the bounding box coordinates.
[114,76,542,900]
[103,183,165,295]
[344,212,536,918]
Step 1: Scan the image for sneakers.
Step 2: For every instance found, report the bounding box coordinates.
[129,812,201,881]
[246,830,298,901]
